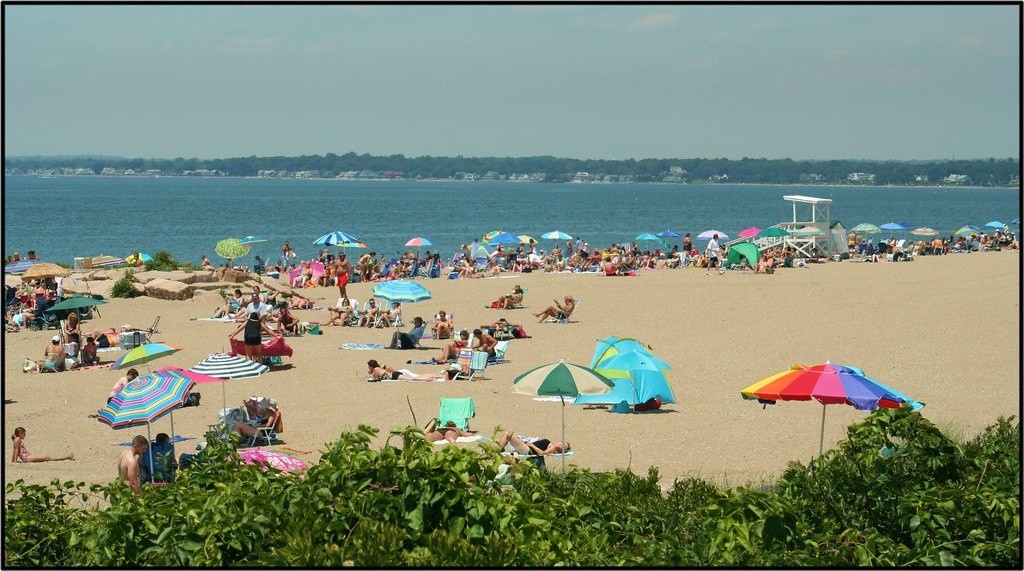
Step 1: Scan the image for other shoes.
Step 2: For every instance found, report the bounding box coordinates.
[718,271,724,275]
[705,272,712,276]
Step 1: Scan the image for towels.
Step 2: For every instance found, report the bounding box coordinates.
[369,368,445,383]
[411,359,505,366]
[501,435,574,457]
[77,363,115,370]
[438,397,475,432]
[197,314,236,322]
[433,436,492,445]
[96,347,122,352]
[337,343,385,350]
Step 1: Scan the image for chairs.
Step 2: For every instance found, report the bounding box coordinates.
[619,259,639,275]
[432,314,509,381]
[39,348,67,373]
[364,300,381,326]
[58,317,80,346]
[245,411,283,447]
[384,300,403,327]
[546,299,580,323]
[398,321,429,348]
[330,298,359,325]
[491,287,528,309]
[435,397,476,432]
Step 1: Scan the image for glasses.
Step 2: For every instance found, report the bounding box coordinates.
[235,292,239,294]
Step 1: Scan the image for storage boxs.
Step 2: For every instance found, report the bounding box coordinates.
[267,272,279,279]
[449,272,458,278]
[119,331,145,349]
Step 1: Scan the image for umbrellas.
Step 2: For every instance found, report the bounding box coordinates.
[4,260,52,273]
[21,262,76,283]
[368,276,433,303]
[81,252,153,267]
[511,357,616,476]
[228,449,308,482]
[96,336,271,483]
[740,359,926,460]
[214,217,1019,266]
[43,292,110,365]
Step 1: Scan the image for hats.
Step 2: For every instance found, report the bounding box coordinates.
[53,335,61,343]
[514,285,520,289]
[252,293,259,299]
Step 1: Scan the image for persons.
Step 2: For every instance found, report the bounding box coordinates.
[329,251,352,298]
[368,309,521,381]
[108,367,139,406]
[118,433,179,493]
[417,420,571,456]
[320,298,405,329]
[10,427,75,462]
[532,295,574,323]
[6,278,60,331]
[200,229,1018,288]
[496,285,524,309]
[227,312,280,365]
[12,251,39,264]
[211,282,311,335]
[87,324,133,348]
[127,252,144,268]
[33,312,101,372]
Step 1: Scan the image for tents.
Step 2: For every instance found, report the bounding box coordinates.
[572,336,677,406]
